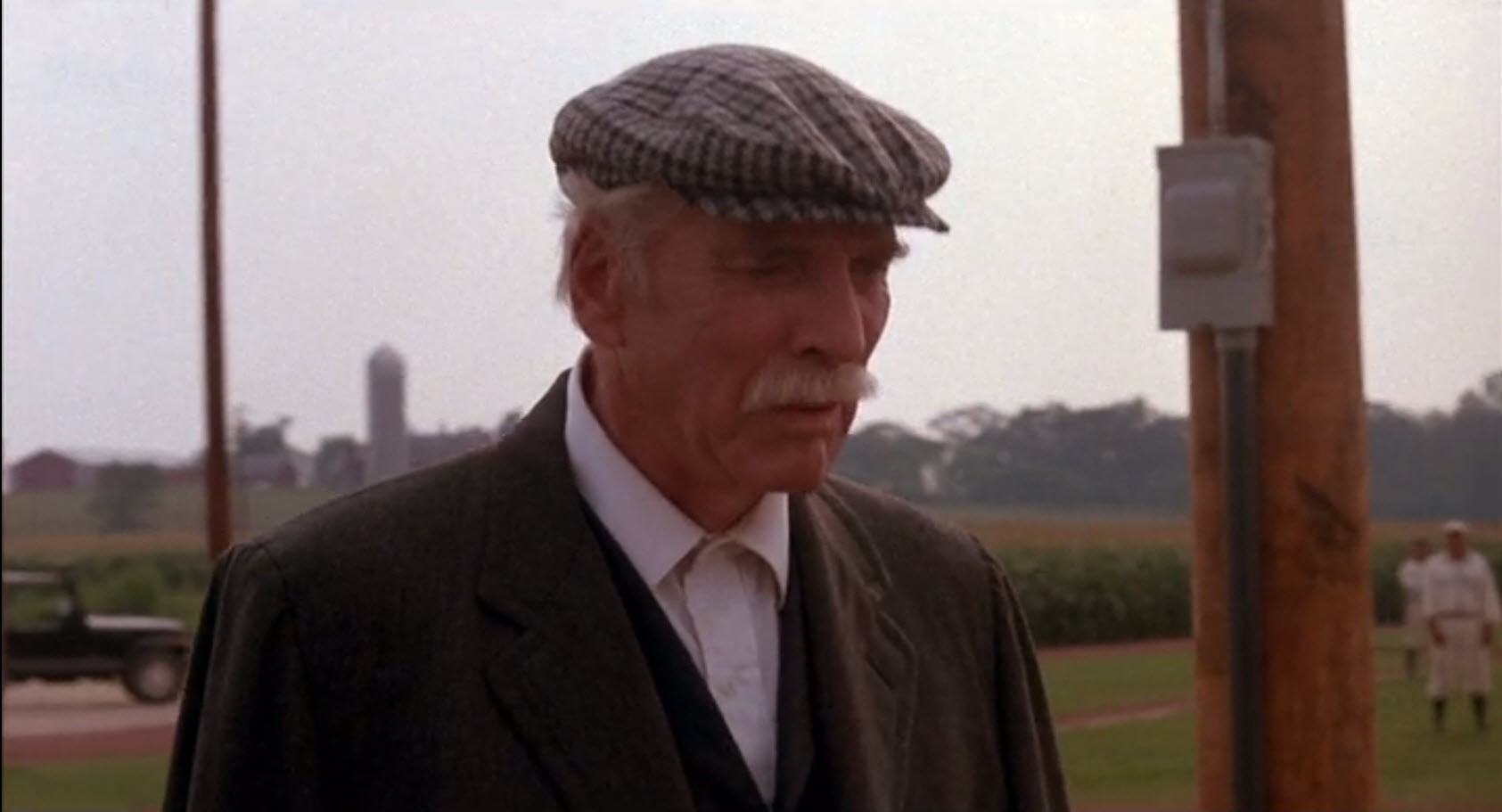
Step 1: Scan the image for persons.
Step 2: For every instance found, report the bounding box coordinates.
[1398,538,1431,683]
[1423,520,1502,733]
[162,42,1070,812]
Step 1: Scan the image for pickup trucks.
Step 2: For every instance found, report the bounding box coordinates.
[2,570,190,706]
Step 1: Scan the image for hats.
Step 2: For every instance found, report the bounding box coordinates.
[1441,519,1467,533]
[551,45,952,235]
